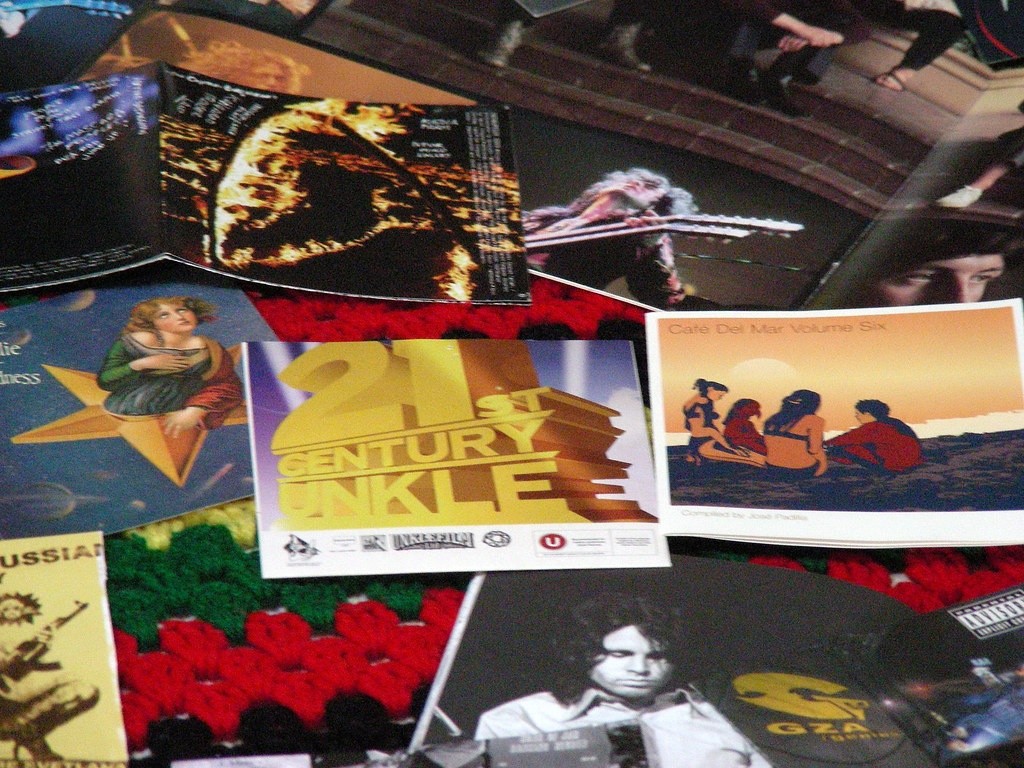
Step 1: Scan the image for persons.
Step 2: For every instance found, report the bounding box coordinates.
[521,168,699,305]
[852,230,1024,308]
[181,41,306,95]
[935,99,1024,210]
[471,593,772,768]
[852,0,969,93]
[483,0,653,73]
[715,0,846,119]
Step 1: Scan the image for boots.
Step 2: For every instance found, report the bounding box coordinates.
[599,23,650,72]
[476,18,522,67]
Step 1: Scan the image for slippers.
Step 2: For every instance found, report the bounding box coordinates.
[876,71,906,93]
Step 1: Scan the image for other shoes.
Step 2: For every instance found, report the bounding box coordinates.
[716,60,811,118]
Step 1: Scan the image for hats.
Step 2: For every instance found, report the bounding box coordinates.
[866,221,1021,271]
